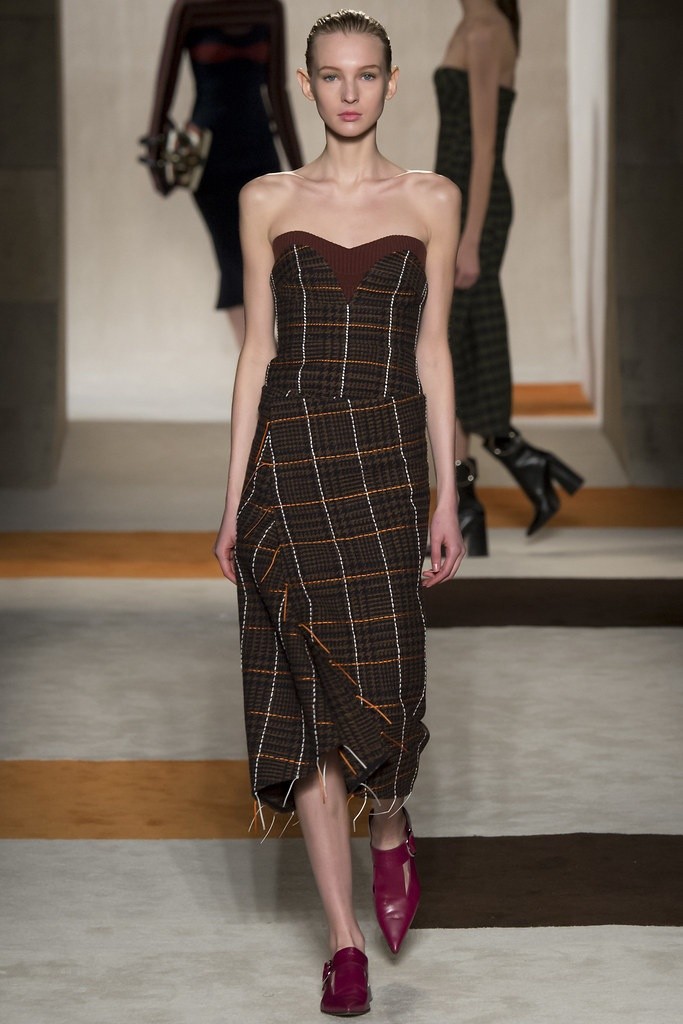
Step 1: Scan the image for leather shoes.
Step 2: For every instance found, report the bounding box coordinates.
[322,943,373,1018]
[367,804,417,957]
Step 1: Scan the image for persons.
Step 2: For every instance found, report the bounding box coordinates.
[423,0,585,556]
[138,0,304,353]
[217,10,465,1017]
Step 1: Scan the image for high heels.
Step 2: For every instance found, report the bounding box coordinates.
[482,426,586,534]
[422,456,488,559]
